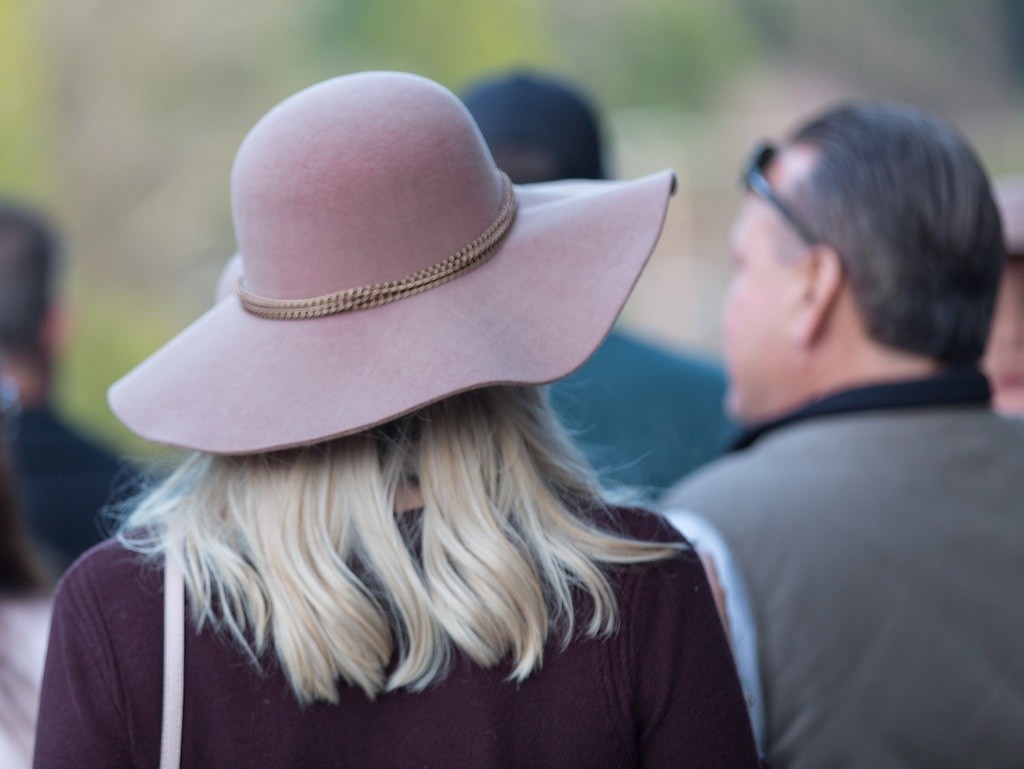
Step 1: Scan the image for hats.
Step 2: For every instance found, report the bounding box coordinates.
[107,69,676,457]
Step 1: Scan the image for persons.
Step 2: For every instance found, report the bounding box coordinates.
[657,99,1024,769]
[454,71,753,511]
[31,72,761,769]
[0,201,175,769]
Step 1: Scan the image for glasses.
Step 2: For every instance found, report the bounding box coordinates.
[734,143,815,245]
